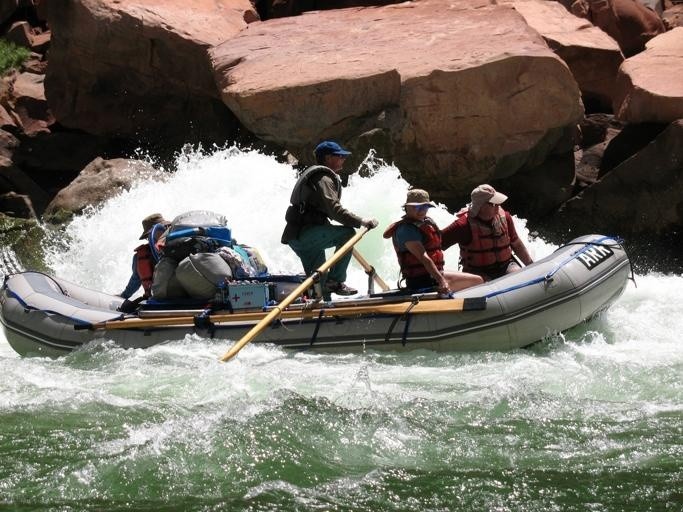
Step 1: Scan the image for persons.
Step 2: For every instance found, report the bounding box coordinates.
[382,189,485,295]
[440,184,534,281]
[115,210,171,300]
[280,141,380,304]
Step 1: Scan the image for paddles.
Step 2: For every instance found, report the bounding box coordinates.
[72,296,487,330]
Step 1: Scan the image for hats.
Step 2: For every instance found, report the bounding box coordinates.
[140,214,171,239]
[471,184,508,205]
[405,189,436,207]
[314,141,352,156]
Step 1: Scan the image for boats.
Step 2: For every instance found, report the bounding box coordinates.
[0,232,632,359]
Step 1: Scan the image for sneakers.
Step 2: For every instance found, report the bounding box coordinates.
[325,280,357,295]
